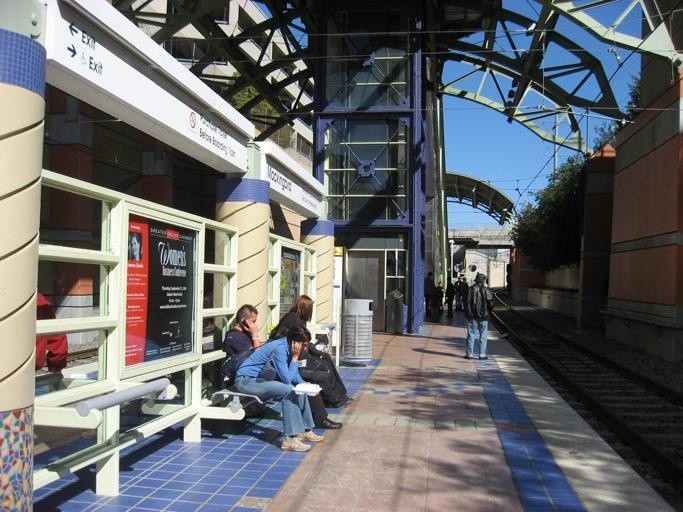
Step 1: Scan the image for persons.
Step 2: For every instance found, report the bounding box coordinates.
[459,277,468,312]
[436,281,444,308]
[453,276,461,312]
[443,277,454,318]
[222,304,347,430]
[266,294,353,408]
[424,271,435,318]
[233,325,326,452]
[462,274,494,360]
[35,289,68,373]
[130,232,140,261]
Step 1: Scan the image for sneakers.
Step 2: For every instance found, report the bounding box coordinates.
[294,430,325,443]
[339,398,354,409]
[463,356,488,360]
[279,437,312,453]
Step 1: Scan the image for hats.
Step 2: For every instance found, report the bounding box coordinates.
[472,274,486,283]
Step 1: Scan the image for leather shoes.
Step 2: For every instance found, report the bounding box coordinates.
[315,418,342,430]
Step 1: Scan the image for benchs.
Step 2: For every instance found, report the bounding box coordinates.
[200,383,273,421]
[140,399,185,415]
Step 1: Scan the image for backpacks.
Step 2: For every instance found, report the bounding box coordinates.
[219,341,276,387]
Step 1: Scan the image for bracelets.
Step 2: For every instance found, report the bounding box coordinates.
[251,338,258,341]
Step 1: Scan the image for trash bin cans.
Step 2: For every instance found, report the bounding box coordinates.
[341,298,373,367]
